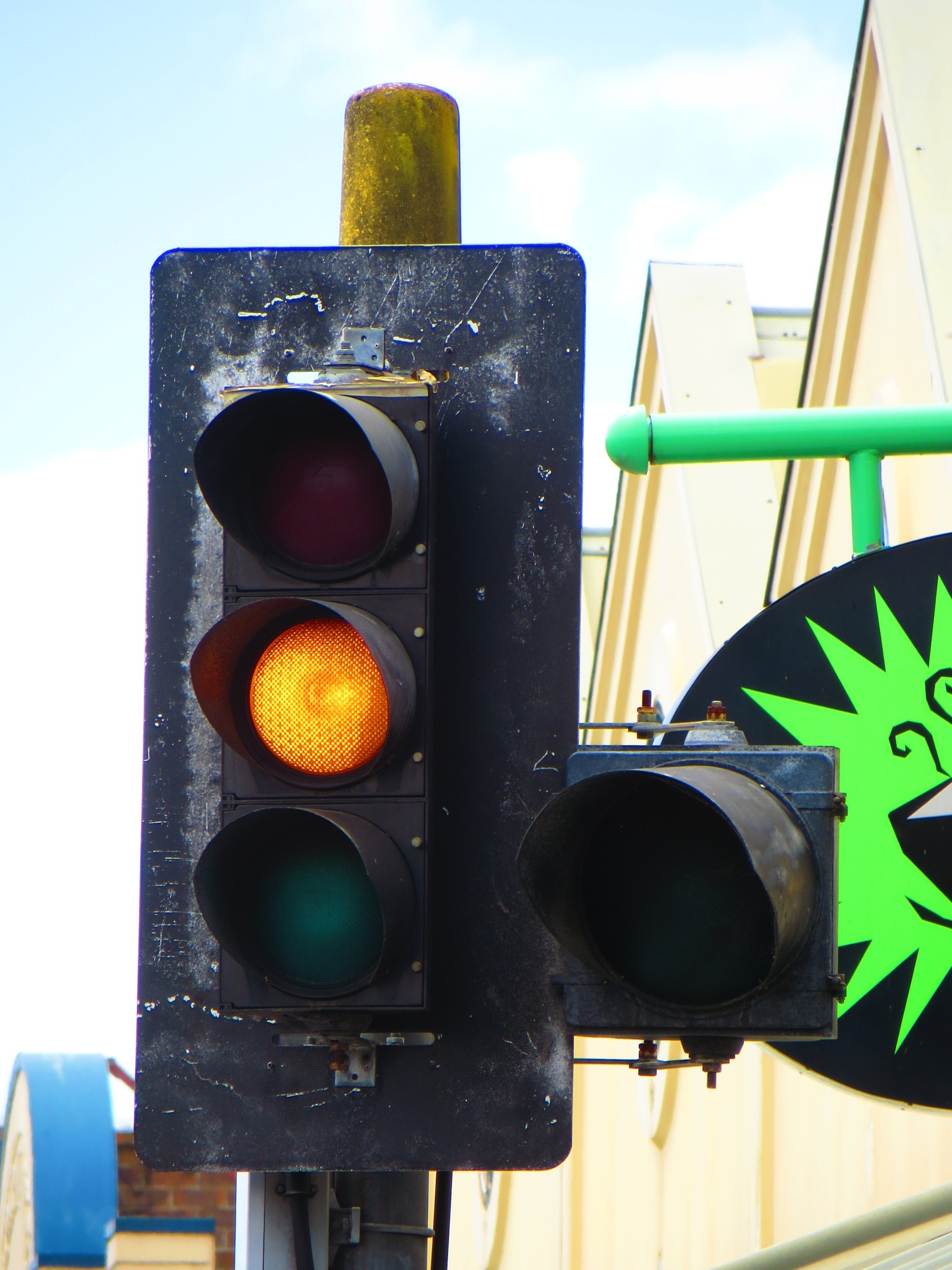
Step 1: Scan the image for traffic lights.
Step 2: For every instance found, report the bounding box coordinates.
[516,748,847,1044]
[136,246,586,1176]
